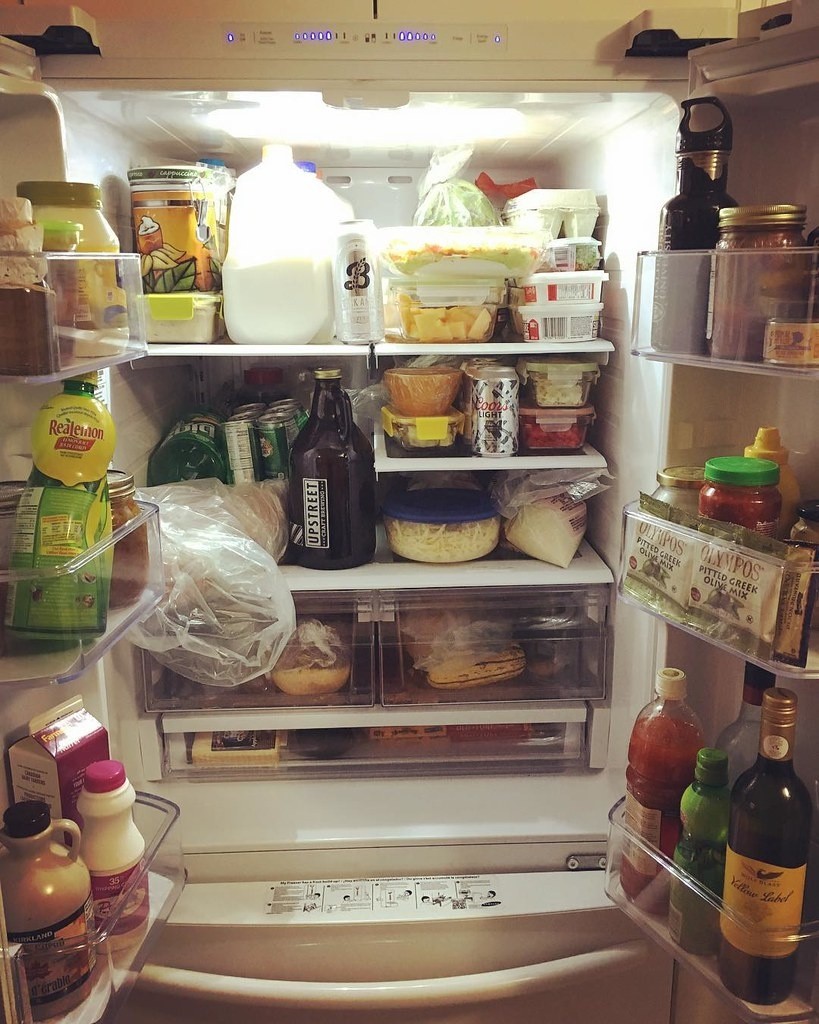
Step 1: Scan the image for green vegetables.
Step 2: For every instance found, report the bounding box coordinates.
[411,179,497,227]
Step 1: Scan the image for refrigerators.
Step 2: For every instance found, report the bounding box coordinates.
[0,6,819,1024]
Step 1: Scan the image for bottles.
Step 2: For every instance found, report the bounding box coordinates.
[0,181,129,375]
[620,667,706,917]
[650,426,819,545]
[287,367,377,570]
[650,96,740,354]
[714,660,776,791]
[0,799,97,1022]
[668,747,732,955]
[75,759,150,956]
[720,687,813,1006]
[2,370,115,642]
[223,139,357,344]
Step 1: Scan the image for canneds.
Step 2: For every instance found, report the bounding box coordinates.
[125,167,219,293]
[645,455,819,629]
[330,219,385,346]
[457,358,518,458]
[219,397,311,485]
[15,179,128,358]
[704,203,809,364]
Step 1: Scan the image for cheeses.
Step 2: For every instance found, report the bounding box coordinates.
[396,294,493,345]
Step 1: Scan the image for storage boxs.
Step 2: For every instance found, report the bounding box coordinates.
[372,590,608,708]
[162,700,589,782]
[140,590,375,714]
[9,694,110,847]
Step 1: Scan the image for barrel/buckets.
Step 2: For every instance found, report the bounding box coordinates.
[127,166,222,300]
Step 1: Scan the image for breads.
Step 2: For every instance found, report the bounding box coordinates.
[267,611,525,695]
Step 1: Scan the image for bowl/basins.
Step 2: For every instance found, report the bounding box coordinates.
[380,403,464,450]
[375,225,552,279]
[380,487,502,564]
[387,278,506,342]
[514,355,602,450]
[132,291,222,343]
[384,367,463,417]
